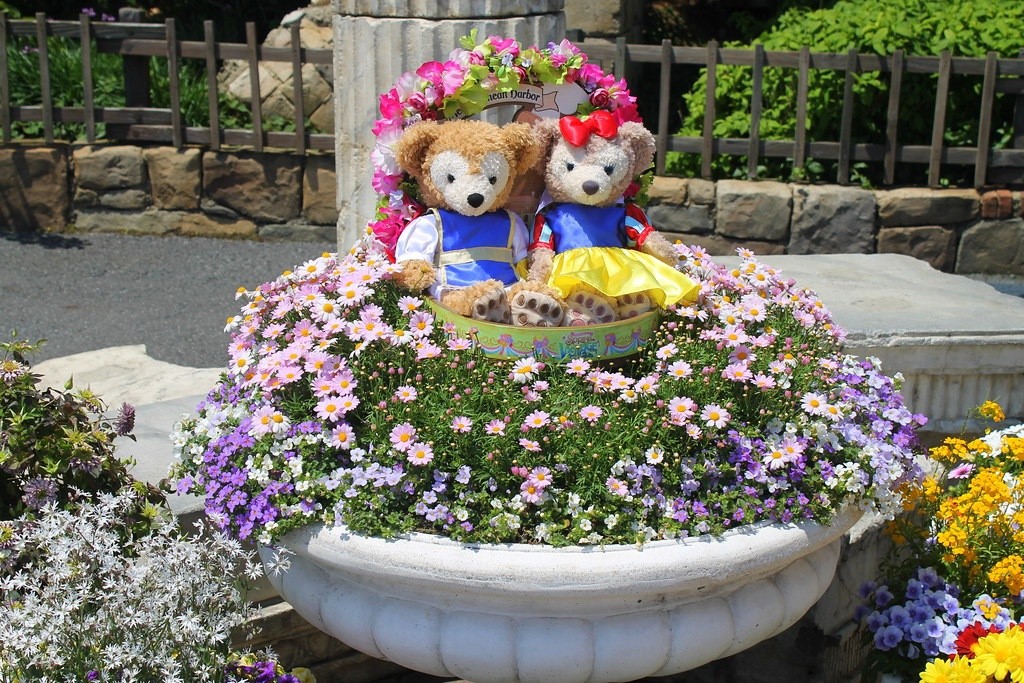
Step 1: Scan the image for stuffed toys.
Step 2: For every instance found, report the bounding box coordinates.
[528,109,702,318]
[392,120,568,327]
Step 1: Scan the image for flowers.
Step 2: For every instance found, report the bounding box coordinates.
[0,33,1024,683]
